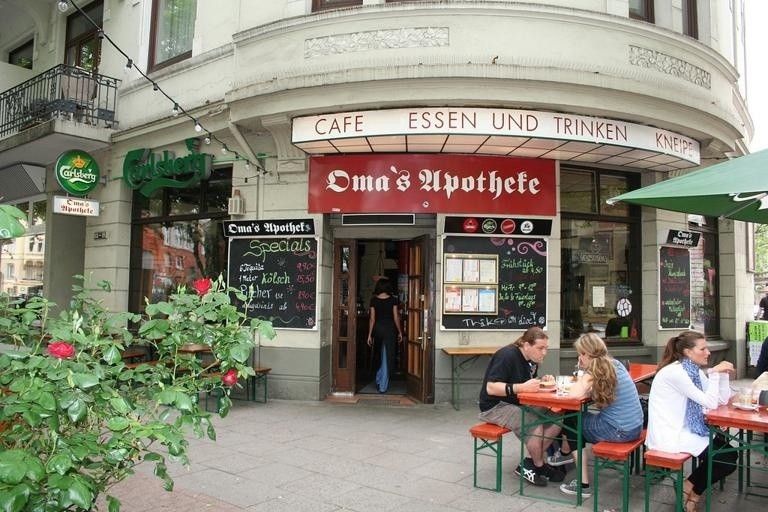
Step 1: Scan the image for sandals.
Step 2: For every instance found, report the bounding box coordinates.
[673,480,691,509]
[684,492,699,512]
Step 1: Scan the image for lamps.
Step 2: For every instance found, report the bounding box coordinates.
[228,190,247,216]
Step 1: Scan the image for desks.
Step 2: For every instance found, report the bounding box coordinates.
[441,346,501,411]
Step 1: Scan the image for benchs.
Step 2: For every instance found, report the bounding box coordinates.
[83,330,273,413]
[592,428,648,511]
[469,424,510,491]
[642,427,730,508]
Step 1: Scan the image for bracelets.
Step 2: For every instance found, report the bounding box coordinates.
[505,384,516,398]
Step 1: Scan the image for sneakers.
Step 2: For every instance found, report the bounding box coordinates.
[560,481,591,498]
[547,447,575,467]
[515,457,547,486]
[539,464,567,483]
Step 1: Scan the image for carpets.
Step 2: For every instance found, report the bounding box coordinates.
[356,398,401,408]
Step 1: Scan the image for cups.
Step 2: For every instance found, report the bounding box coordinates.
[535,364,583,399]
[737,387,753,408]
[622,327,628,338]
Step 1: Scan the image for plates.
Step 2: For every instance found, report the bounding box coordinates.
[730,403,760,412]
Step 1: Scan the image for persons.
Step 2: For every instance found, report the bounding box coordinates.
[367,278,404,393]
[643,331,739,512]
[479,327,563,488]
[754,283,768,320]
[546,332,644,499]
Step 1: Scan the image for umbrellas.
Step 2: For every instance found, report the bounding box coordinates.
[606,150,768,224]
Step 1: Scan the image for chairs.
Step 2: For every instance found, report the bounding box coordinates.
[60,73,96,124]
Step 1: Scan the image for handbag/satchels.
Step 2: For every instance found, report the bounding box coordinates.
[640,398,652,428]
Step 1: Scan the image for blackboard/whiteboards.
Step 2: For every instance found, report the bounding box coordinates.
[439,233,550,332]
[226,235,320,331]
[657,245,692,330]
